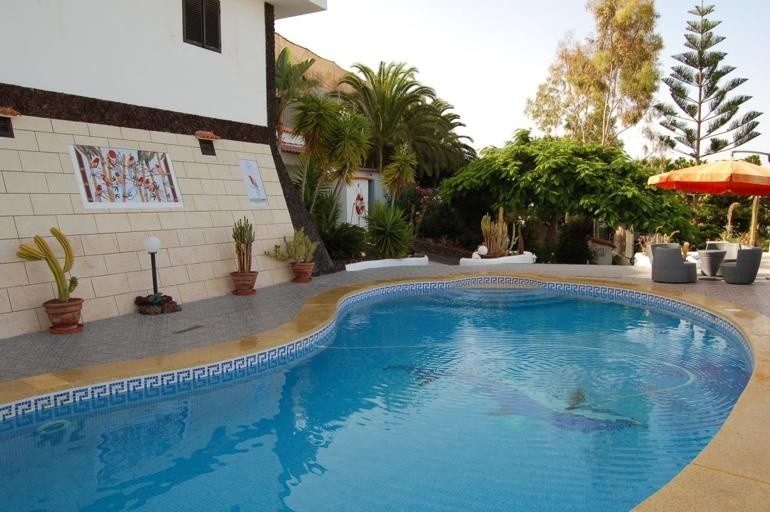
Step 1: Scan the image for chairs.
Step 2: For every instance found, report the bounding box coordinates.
[651,246,697,284]
[649,243,681,264]
[701,241,741,276]
[719,247,763,285]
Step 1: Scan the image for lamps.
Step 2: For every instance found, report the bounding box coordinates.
[0,107,22,117]
[195,130,220,141]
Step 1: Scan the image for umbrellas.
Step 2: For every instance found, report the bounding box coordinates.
[648,151,770,196]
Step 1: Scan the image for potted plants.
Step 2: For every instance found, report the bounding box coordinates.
[15,227,85,335]
[230,214,259,295]
[261,227,321,283]
